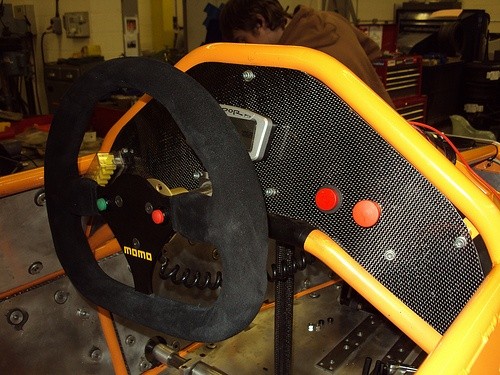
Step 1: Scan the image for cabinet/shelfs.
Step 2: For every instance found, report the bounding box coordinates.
[44,56,104,117]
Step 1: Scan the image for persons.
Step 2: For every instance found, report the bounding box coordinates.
[219,0,398,112]
